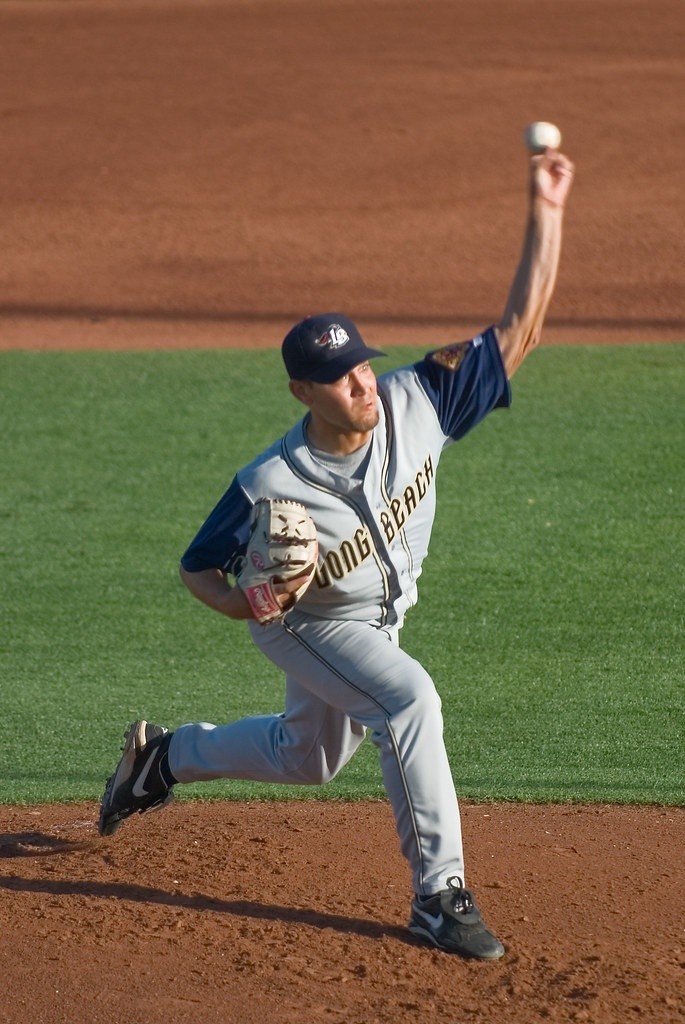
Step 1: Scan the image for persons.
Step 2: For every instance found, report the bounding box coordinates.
[96,146,574,961]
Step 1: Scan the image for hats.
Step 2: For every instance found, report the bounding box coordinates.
[281,313,388,384]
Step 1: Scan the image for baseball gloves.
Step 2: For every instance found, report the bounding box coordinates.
[232,496,318,630]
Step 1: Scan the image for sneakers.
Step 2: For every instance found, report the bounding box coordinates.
[93,720,176,837]
[407,876,505,961]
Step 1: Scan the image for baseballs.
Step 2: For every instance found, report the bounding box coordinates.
[524,121,562,153]
